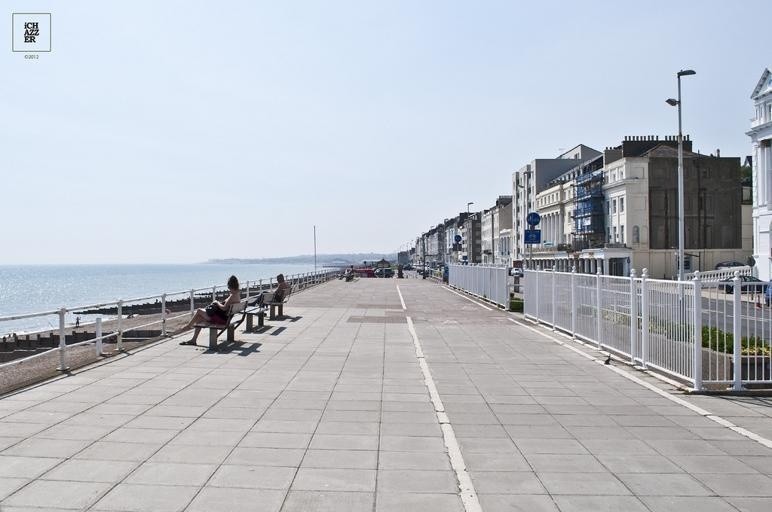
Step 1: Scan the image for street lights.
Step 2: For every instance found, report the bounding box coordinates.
[468,202,474,266]
[666,68,696,300]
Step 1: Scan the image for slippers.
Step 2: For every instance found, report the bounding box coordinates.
[180,341,196,345]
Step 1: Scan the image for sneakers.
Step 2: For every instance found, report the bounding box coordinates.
[248,303,255,306]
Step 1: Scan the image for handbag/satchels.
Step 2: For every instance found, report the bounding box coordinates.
[207,302,230,317]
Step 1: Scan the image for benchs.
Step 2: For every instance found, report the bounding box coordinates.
[193,288,292,350]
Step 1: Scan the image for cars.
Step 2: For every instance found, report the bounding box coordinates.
[719,275,769,294]
[716,260,745,270]
[355,262,448,280]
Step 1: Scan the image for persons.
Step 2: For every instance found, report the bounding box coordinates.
[181,275,241,346]
[247,274,289,308]
